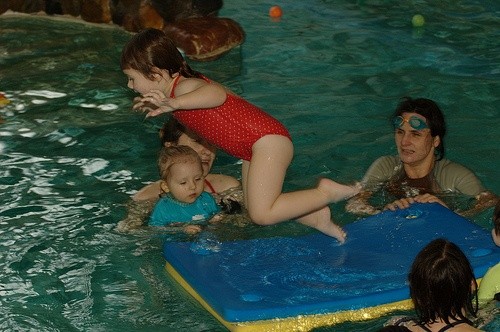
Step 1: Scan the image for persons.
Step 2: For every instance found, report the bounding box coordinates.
[472,198,500,309]
[117,29,363,245]
[346,96,500,218]
[380,240,500,332]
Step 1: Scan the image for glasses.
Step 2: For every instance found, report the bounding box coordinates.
[393,115,430,130]
